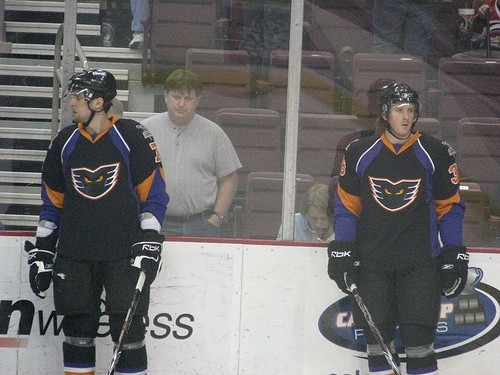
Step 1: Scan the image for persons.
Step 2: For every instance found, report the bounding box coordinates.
[275,183,335,243]
[29,69,170,375]
[370,0,500,62]
[327,79,470,374]
[241,0,287,109]
[129,0,151,49]
[139,70,243,238]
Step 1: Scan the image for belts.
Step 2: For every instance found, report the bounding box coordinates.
[165,208,213,223]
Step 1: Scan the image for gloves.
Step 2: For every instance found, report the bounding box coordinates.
[327,240,363,294]
[25,240,56,299]
[130,232,165,289]
[438,254,469,299]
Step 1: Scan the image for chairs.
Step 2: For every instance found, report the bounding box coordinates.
[142,0,500,248]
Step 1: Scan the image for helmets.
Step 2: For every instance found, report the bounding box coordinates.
[68,70,116,101]
[379,83,418,113]
[369,78,394,94]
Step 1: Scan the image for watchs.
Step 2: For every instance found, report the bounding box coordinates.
[211,211,226,224]
